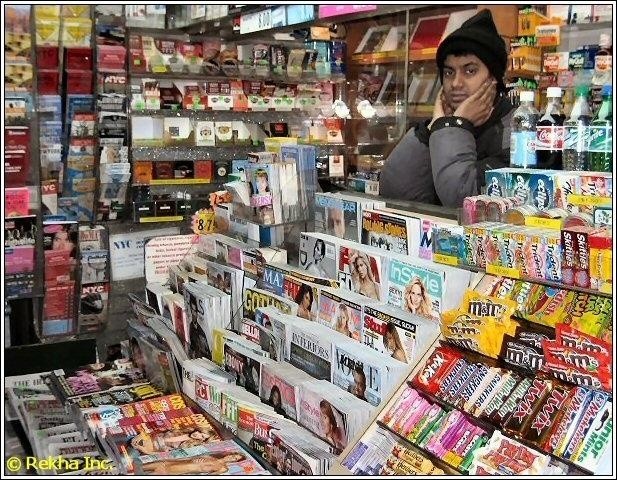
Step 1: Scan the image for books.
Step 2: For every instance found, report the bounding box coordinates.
[5,5,476,476]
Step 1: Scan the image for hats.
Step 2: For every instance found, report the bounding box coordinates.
[435,8,508,83]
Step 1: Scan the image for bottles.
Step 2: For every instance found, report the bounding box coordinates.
[590,33,611,84]
[509,85,613,172]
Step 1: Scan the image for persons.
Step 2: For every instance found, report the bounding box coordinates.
[379,8,518,210]
